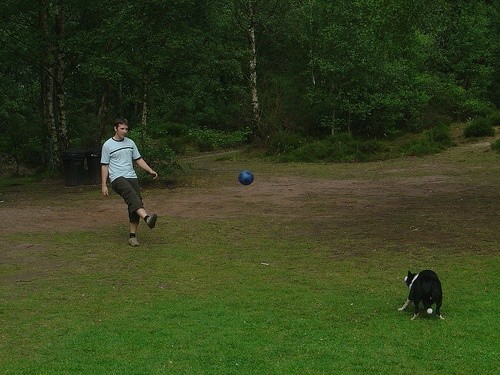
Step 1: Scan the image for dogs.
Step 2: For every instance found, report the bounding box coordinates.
[397,268,445,322]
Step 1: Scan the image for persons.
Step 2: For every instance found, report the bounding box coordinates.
[101,118,157,246]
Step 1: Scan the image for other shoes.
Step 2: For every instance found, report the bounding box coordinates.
[129,238,140,247]
[147,214,157,228]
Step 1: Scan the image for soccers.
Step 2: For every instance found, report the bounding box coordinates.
[238,170,254,186]
